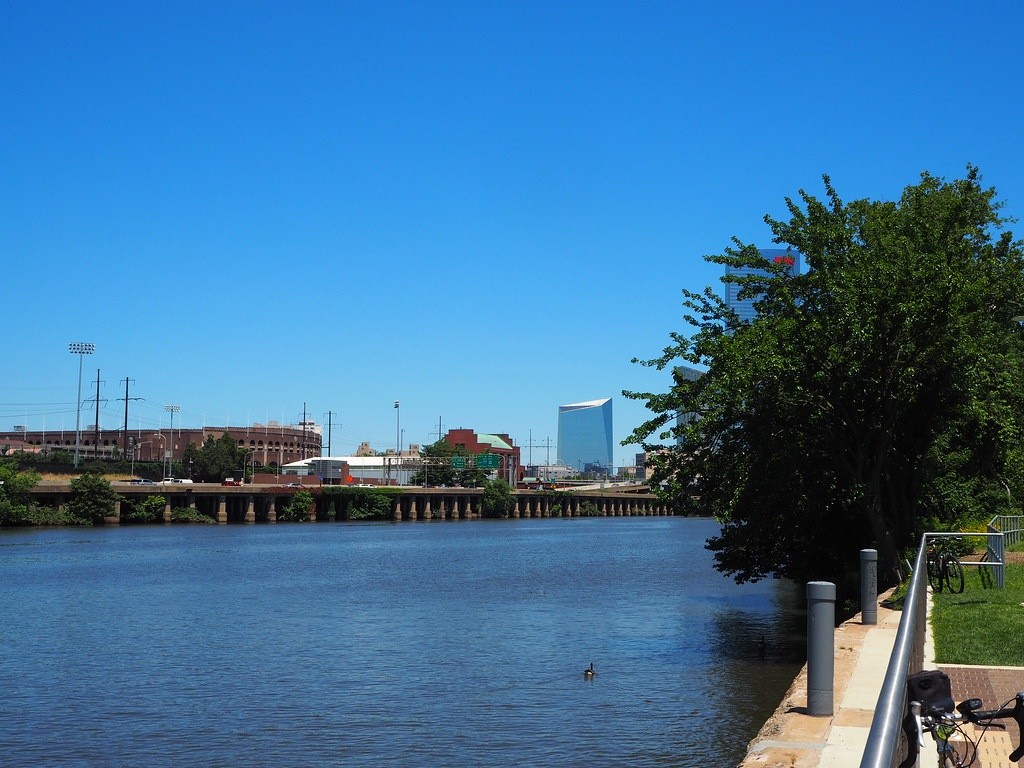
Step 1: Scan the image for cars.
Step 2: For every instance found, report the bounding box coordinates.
[283,483,305,487]
[221,481,241,486]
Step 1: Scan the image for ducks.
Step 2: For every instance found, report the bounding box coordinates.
[584,662,594,674]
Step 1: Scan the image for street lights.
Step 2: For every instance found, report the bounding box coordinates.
[489,453,505,478]
[554,459,565,493]
[154,434,166,485]
[164,405,181,476]
[68,342,95,468]
[418,450,427,488]
[303,442,322,472]
[243,451,253,483]
[132,441,152,475]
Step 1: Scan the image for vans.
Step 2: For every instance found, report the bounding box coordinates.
[171,479,193,483]
[356,484,377,488]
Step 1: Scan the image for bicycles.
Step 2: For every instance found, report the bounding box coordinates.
[898,692,1024,768]
[927,536,964,594]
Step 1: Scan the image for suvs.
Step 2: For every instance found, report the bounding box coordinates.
[160,478,174,483]
[130,479,157,485]
